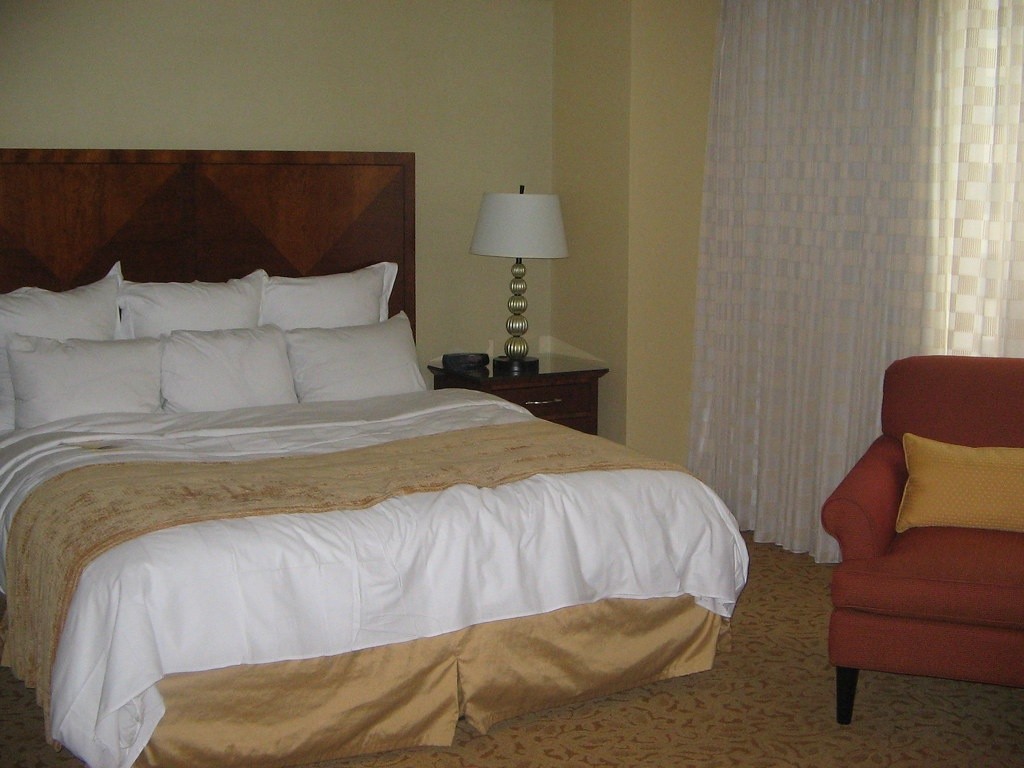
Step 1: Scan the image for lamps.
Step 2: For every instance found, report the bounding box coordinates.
[469,184,570,375]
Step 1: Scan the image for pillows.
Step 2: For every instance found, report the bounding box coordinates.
[0,261,428,432]
[895,433,1024,534]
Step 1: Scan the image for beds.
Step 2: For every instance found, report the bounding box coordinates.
[0,386,750,768]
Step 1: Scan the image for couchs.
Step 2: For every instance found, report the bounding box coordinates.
[820,355,1024,727]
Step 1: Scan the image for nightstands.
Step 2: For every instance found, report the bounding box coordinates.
[427,352,610,435]
